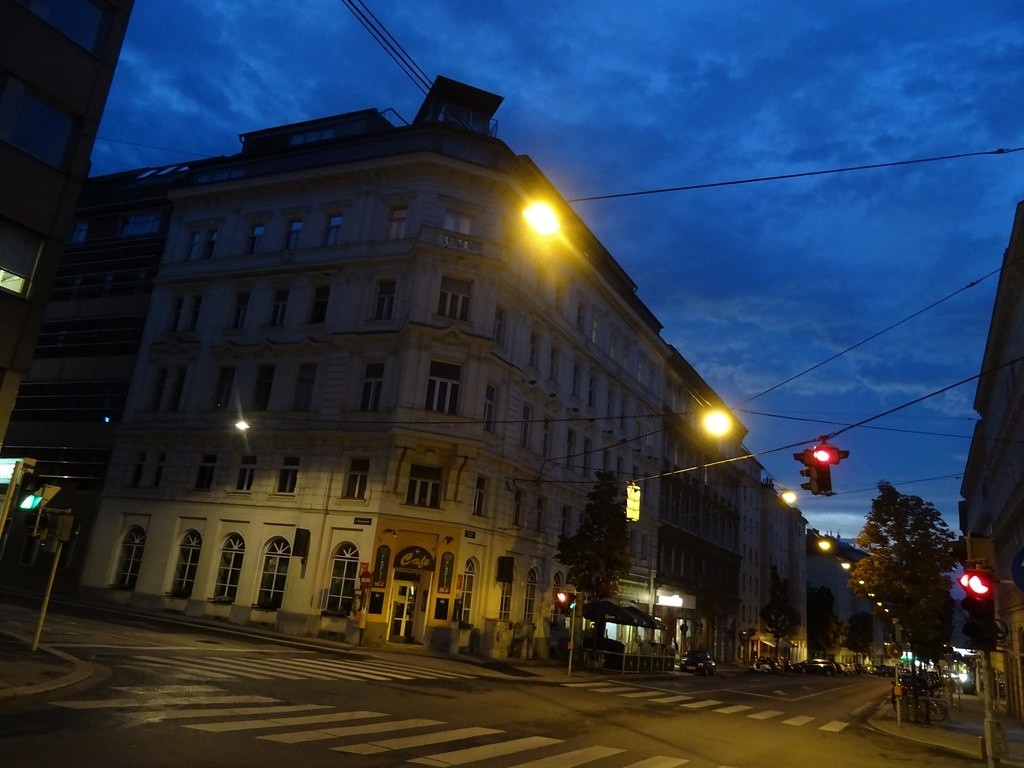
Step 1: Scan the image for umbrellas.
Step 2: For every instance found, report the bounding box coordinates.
[581,600,670,654]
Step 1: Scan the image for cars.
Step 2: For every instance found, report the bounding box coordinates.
[890,671,943,693]
[680,649,717,676]
[805,656,837,677]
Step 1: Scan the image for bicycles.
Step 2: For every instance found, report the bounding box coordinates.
[894,694,950,723]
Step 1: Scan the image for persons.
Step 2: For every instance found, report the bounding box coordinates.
[669,638,678,652]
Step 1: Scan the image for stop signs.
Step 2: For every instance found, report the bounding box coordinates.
[360,571,372,589]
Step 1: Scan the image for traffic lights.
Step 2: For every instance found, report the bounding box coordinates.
[956,571,996,650]
[793,445,849,495]
[15,471,44,515]
[555,591,577,616]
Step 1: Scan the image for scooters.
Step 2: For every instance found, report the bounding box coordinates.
[836,661,894,676]
[750,655,802,675]
